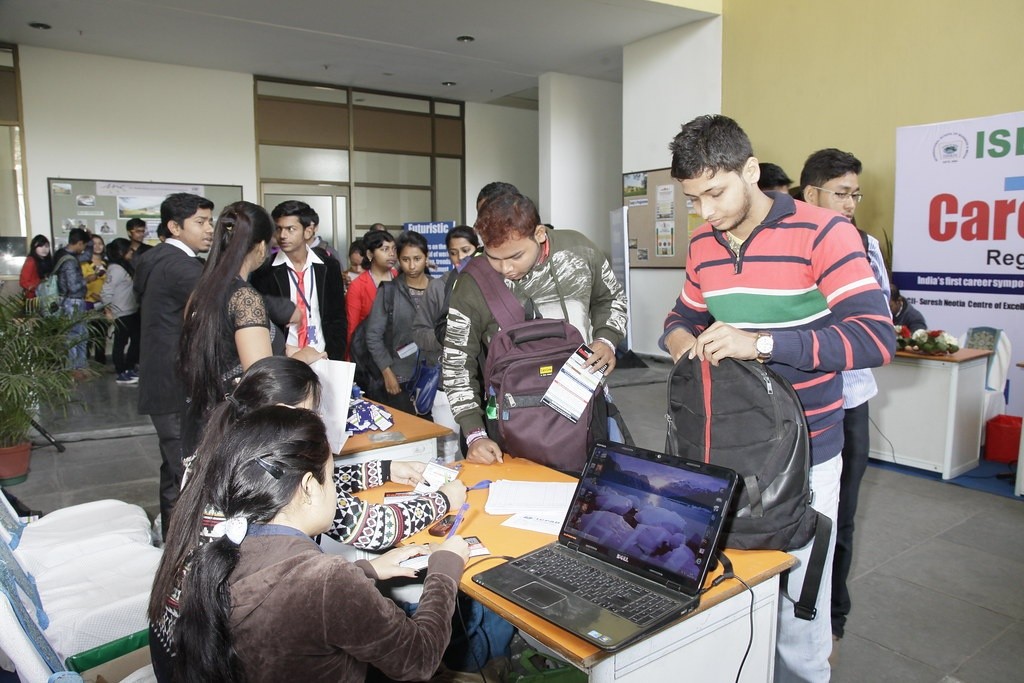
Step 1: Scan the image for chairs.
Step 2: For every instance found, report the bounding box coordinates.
[957,329,1011,445]
[0,490,168,683]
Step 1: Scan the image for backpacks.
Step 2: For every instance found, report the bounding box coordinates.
[350,280,394,404]
[457,256,608,476]
[35,254,76,323]
[665,343,817,550]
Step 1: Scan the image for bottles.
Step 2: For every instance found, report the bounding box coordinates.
[486,386,498,420]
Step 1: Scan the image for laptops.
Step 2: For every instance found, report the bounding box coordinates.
[471,437,740,653]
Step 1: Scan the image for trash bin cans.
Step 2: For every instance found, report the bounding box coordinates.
[985,414,1023,463]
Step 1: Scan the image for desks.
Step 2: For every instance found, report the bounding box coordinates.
[344,452,799,682]
[869,348,994,483]
[334,395,453,494]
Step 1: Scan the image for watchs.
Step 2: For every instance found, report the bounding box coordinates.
[753,331,774,360]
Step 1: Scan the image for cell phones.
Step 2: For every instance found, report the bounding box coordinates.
[429,514,465,537]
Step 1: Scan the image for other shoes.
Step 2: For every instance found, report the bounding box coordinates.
[72,366,94,379]
[116,372,137,384]
[126,369,139,382]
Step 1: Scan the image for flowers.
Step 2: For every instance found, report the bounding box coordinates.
[895,324,953,357]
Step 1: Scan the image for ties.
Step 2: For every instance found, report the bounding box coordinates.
[291,268,308,348]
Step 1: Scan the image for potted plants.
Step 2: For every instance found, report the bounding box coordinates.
[1,290,124,480]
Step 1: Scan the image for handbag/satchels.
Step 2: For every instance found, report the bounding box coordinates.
[394,594,513,671]
[507,649,588,683]
[409,360,441,415]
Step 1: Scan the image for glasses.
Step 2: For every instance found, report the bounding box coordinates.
[811,186,863,204]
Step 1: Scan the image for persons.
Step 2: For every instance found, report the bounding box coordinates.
[247,200,348,361]
[132,194,303,543]
[20,217,166,384]
[175,404,470,683]
[413,225,479,433]
[888,283,927,335]
[434,182,628,480]
[366,230,434,419]
[178,201,327,458]
[799,150,893,640]
[756,163,794,194]
[657,114,897,683]
[146,356,467,683]
[341,222,399,363]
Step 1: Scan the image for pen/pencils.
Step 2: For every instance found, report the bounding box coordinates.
[446,504,469,539]
